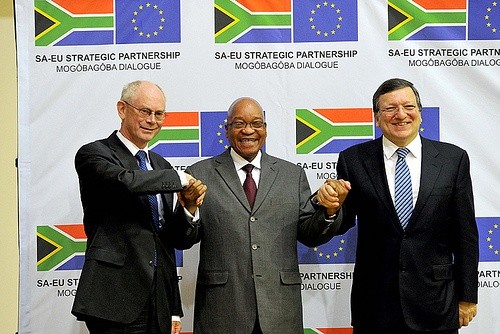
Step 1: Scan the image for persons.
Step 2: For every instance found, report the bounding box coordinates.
[70,79,206,333]
[174,95,349,333]
[308,77,480,333]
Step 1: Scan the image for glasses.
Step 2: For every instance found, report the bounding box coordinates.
[122,100,168,121]
[227,121,264,129]
[378,103,419,115]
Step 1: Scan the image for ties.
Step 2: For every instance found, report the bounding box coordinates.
[241,164,257,209]
[135,150,159,267]
[395,148,413,232]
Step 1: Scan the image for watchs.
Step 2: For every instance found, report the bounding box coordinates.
[326,212,339,220]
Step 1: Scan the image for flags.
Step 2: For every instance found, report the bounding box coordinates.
[34,0,113,46]
[214,0,291,44]
[375,107,440,143]
[147,110,198,157]
[201,111,266,157]
[296,108,374,154]
[469,1,500,40]
[297,220,357,265]
[474,217,500,262]
[389,1,466,41]
[116,0,181,45]
[175,248,183,267]
[37,224,88,271]
[294,0,359,43]
